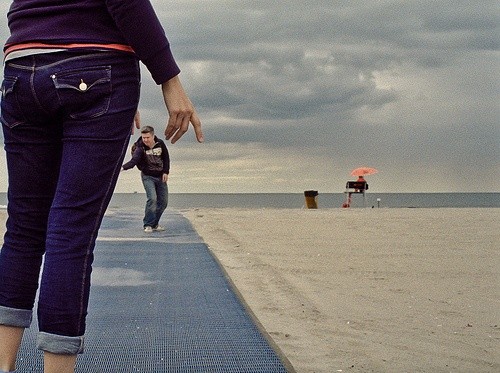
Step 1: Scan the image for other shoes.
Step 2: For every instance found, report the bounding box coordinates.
[144,225,153,233]
[152,225,165,231]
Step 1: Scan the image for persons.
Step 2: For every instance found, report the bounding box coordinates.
[119,126,170,233]
[1,1,203,373]
[355,176,366,193]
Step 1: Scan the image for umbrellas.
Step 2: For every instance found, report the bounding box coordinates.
[352,167,378,176]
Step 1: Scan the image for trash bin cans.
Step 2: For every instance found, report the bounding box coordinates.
[304,190,319,209]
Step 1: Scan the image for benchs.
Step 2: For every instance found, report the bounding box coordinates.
[344,181,368,208]
